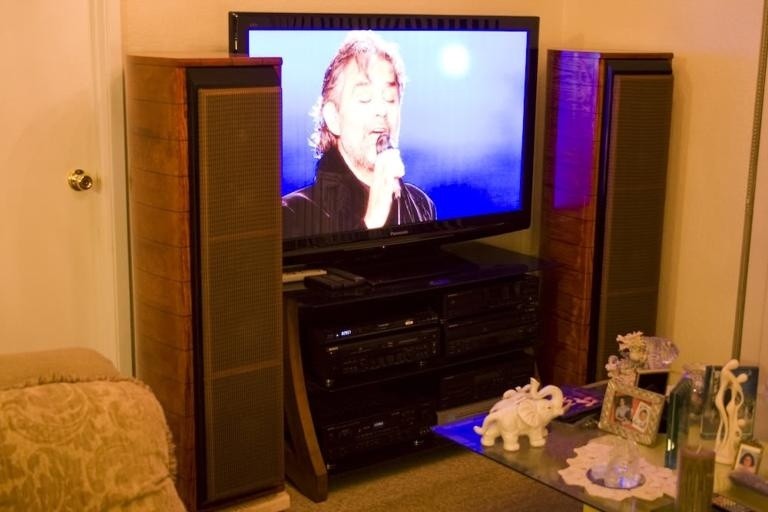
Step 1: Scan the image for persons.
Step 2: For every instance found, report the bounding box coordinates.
[726,373,749,449]
[616,397,631,425]
[714,359,739,447]
[282,41,437,239]
[740,453,755,474]
[634,409,648,429]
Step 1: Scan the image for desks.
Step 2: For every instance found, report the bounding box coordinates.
[423,377,768,510]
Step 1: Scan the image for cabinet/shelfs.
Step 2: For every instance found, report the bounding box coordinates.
[278,237,543,503]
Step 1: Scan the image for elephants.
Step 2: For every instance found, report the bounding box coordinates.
[472,377,572,453]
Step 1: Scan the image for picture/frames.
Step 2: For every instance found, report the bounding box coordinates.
[730,435,764,478]
[594,370,692,469]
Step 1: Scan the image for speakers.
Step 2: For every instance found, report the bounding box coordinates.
[124,52,288,512]
[540,49,675,389]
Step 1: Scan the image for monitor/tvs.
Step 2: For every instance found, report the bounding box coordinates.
[228,9,540,287]
